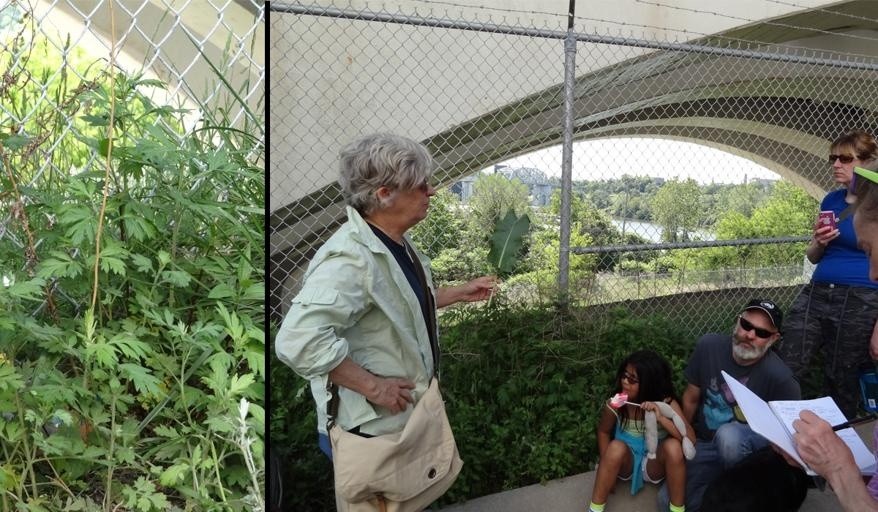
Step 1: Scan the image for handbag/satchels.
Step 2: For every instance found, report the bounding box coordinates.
[327,376,465,512]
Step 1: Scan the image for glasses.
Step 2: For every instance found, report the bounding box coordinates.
[829,154,854,164]
[738,314,774,338]
[420,177,429,193]
[851,166,878,195]
[622,372,639,384]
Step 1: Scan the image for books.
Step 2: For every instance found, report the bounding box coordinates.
[721,370,878,478]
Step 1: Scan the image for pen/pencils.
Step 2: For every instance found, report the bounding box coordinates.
[832,414,875,432]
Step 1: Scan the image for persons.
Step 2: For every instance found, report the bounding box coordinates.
[654,298,809,512]
[765,157,878,512]
[587,348,698,512]
[273,129,501,512]
[771,130,878,423]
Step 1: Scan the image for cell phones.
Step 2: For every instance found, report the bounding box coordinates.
[819,211,836,240]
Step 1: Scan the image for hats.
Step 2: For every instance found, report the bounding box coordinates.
[745,299,783,327]
